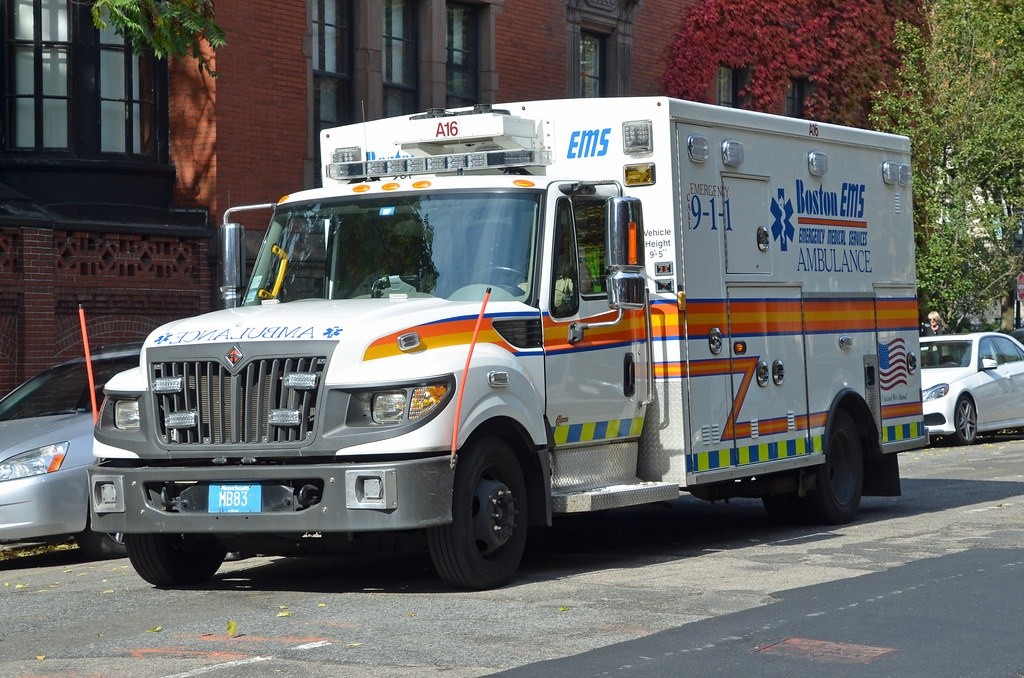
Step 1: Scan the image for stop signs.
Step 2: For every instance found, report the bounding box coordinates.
[1016,272,1024,302]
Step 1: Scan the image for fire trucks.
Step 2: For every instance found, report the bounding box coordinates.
[85,96,931,593]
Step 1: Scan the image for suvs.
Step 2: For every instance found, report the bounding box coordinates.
[0,340,259,563]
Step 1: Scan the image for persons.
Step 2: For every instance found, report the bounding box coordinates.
[347,219,447,300]
[512,246,576,312]
[921,310,947,335]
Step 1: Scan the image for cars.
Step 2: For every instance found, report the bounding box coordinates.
[919,328,1024,445]
